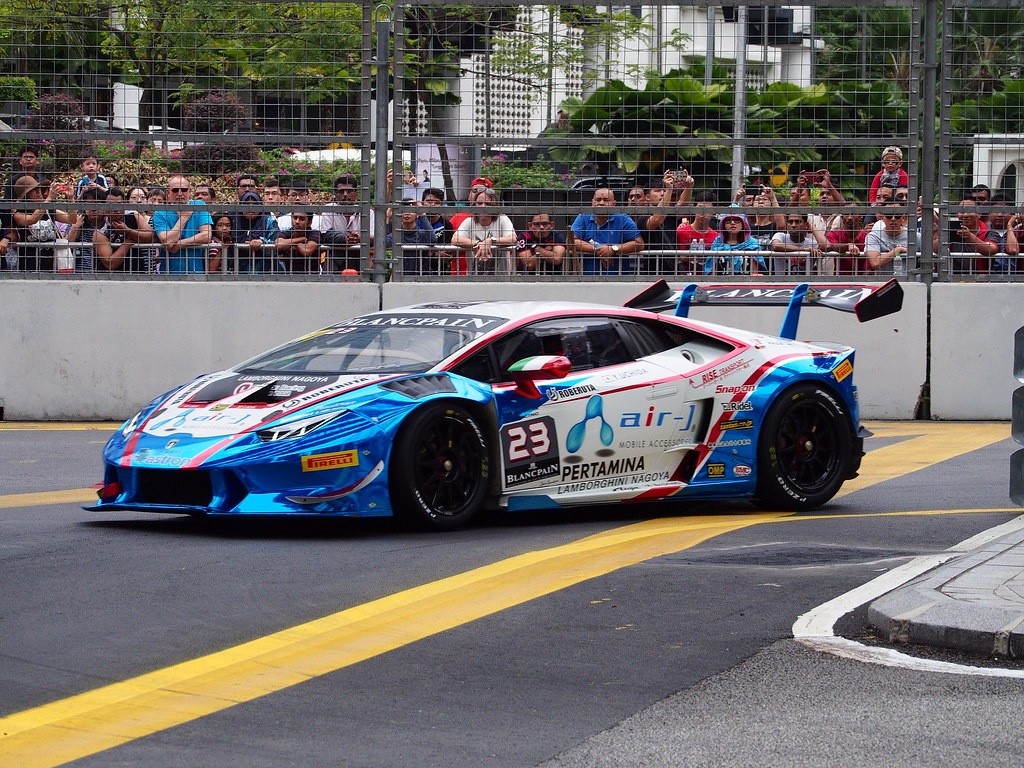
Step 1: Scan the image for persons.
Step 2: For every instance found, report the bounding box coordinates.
[423,169,430,183]
[0,145,1024,275]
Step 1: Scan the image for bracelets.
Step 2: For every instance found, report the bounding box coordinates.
[3,236,11,241]
[474,240,480,248]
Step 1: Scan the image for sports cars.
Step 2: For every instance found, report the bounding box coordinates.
[78,274,905,535]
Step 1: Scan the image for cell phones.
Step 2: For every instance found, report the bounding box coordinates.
[804,173,820,183]
[57,183,68,191]
[401,171,410,181]
[743,185,763,195]
[948,221,963,229]
[669,171,687,183]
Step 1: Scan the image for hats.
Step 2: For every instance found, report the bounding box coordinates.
[882,146,902,159]
[471,178,492,189]
[15,176,40,201]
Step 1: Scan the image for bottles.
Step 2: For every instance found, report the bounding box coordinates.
[589,239,609,262]
[691,238,704,265]
[892,253,902,275]
[717,255,726,275]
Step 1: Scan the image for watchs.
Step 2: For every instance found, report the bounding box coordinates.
[490,237,496,245]
[531,244,537,250]
[611,245,619,254]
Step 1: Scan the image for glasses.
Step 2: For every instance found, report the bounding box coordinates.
[531,221,551,227]
[239,186,256,190]
[477,186,496,195]
[724,218,741,224]
[130,196,146,201]
[337,189,355,194]
[171,188,188,193]
[885,214,903,219]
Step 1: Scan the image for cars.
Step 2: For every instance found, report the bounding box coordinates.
[149,125,207,158]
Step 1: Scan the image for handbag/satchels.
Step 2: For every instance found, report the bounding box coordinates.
[52,238,74,273]
